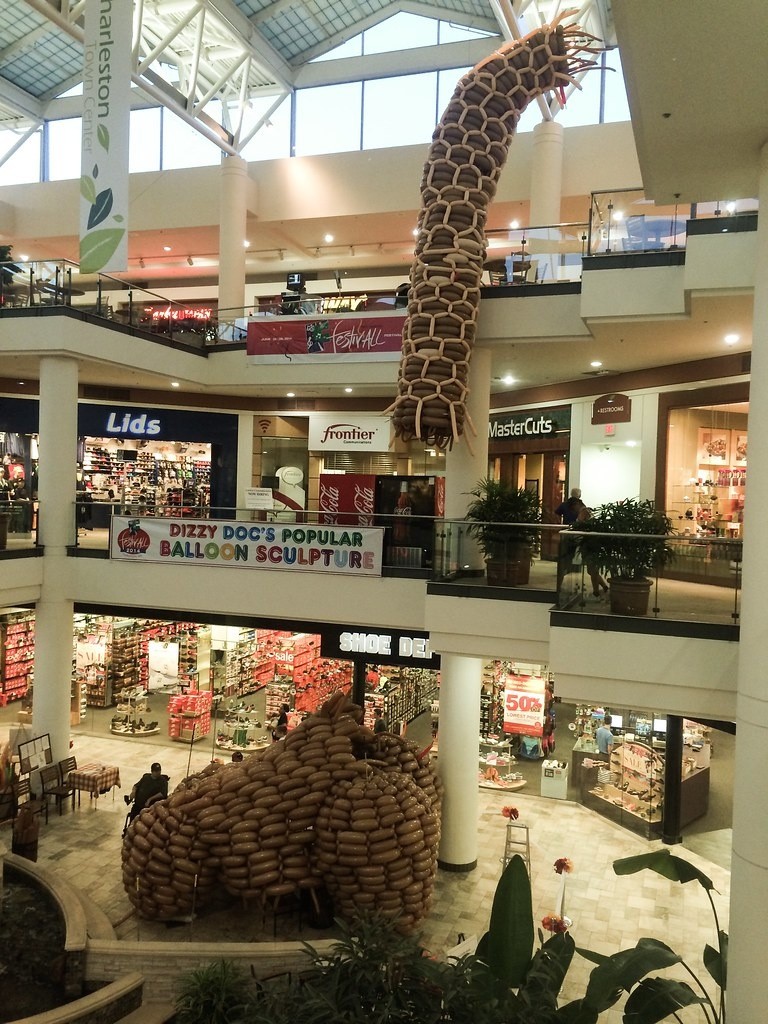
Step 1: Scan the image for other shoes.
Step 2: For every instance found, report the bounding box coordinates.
[108,621,196,699]
[110,715,158,734]
[276,660,355,693]
[2,621,34,698]
[593,748,664,819]
[584,593,601,603]
[228,634,274,694]
[599,588,609,595]
[72,661,106,717]
[218,702,271,750]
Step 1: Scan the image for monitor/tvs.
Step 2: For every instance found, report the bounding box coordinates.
[609,715,622,731]
[261,476,279,489]
[653,718,667,736]
[288,273,301,284]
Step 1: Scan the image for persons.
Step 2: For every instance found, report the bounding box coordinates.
[267,702,290,742]
[0,451,33,550]
[594,714,615,771]
[209,751,244,765]
[553,487,612,603]
[372,708,388,735]
[130,762,169,828]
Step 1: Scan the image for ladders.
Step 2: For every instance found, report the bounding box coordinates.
[501,822,531,888]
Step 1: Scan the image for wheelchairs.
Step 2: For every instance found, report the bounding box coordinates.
[121,773,170,839]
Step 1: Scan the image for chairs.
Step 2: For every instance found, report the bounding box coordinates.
[39,764,76,816]
[96,296,109,319]
[11,779,48,829]
[13,289,30,307]
[35,278,60,306]
[58,758,92,808]
[625,213,665,250]
[526,262,548,285]
[622,237,648,251]
[101,306,120,323]
[499,251,532,285]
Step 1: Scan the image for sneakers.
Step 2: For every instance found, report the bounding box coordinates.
[477,733,523,787]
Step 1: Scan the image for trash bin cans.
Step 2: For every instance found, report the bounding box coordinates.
[0,513,13,550]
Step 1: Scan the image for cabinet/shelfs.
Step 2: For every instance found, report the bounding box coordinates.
[670,483,719,558]
[216,708,270,754]
[479,741,528,792]
[109,696,160,737]
[0,620,35,704]
[82,451,112,474]
[84,665,113,710]
[108,452,211,518]
[264,682,295,720]
[237,629,280,699]
[480,695,492,738]
[112,620,197,706]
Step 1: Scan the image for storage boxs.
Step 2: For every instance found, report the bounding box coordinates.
[0,621,438,738]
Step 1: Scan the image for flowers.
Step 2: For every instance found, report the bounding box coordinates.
[541,911,567,937]
[553,857,573,921]
[502,806,519,853]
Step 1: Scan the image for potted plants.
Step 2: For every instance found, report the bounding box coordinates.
[570,495,680,616]
[461,476,530,588]
[510,485,549,585]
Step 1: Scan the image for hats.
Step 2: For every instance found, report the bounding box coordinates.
[150,763,161,772]
[210,758,224,764]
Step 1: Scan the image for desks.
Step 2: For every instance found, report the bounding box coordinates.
[631,219,686,248]
[483,259,531,285]
[631,240,665,252]
[50,286,85,307]
[65,762,122,812]
[115,309,138,325]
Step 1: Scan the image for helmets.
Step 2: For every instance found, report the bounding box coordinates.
[232,752,243,761]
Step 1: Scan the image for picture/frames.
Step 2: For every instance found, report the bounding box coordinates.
[697,427,731,466]
[730,429,748,466]
